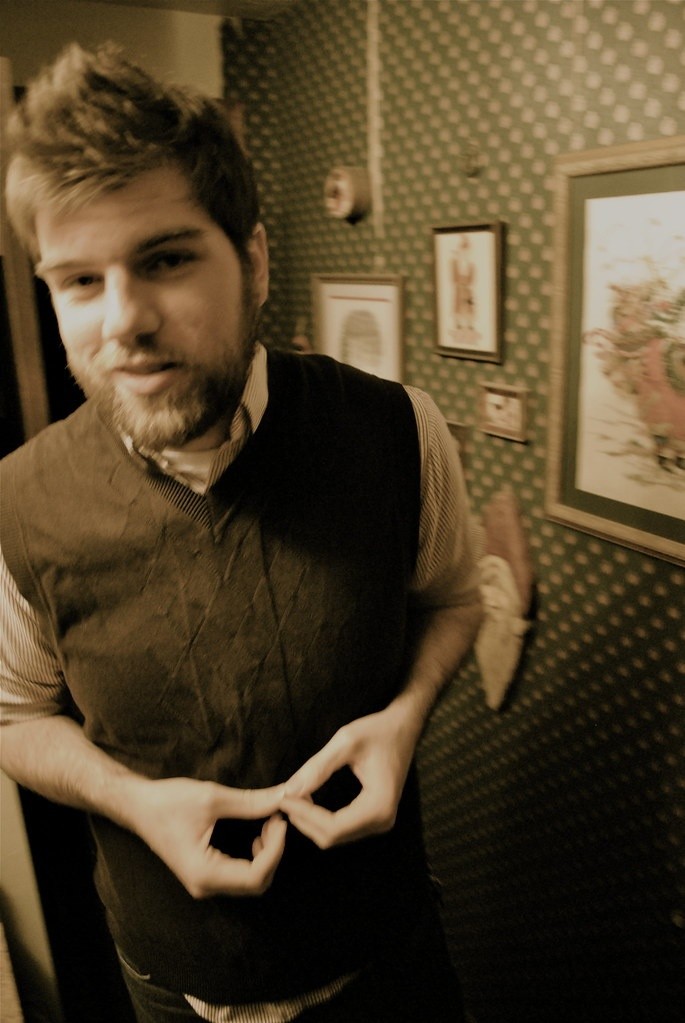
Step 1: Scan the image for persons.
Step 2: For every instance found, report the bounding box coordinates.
[0,47,485,1023]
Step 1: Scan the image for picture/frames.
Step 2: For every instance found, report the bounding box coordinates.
[477,381,532,442]
[542,134,685,568]
[311,272,409,384]
[431,220,507,363]
